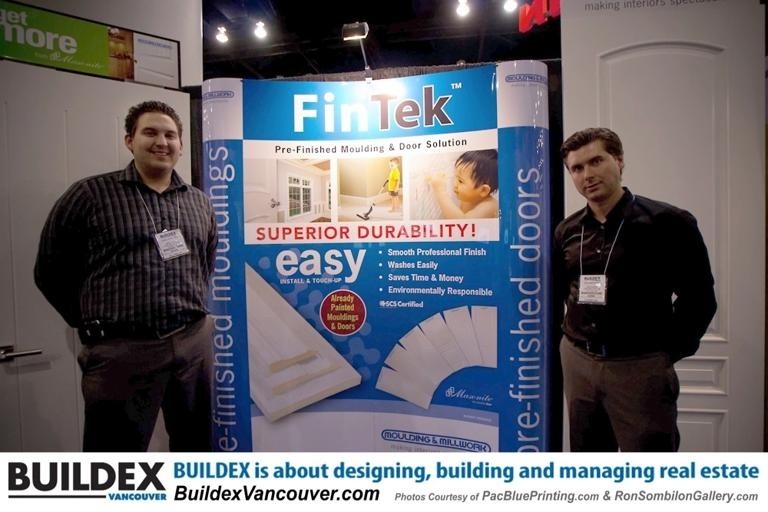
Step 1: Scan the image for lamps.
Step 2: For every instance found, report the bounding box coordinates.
[341,20,372,77]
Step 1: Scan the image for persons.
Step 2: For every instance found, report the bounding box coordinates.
[427,149,498,220]
[31,101,220,452]
[387,157,402,212]
[551,126,717,453]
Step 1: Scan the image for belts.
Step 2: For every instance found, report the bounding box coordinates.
[563,332,660,357]
[142,322,193,339]
